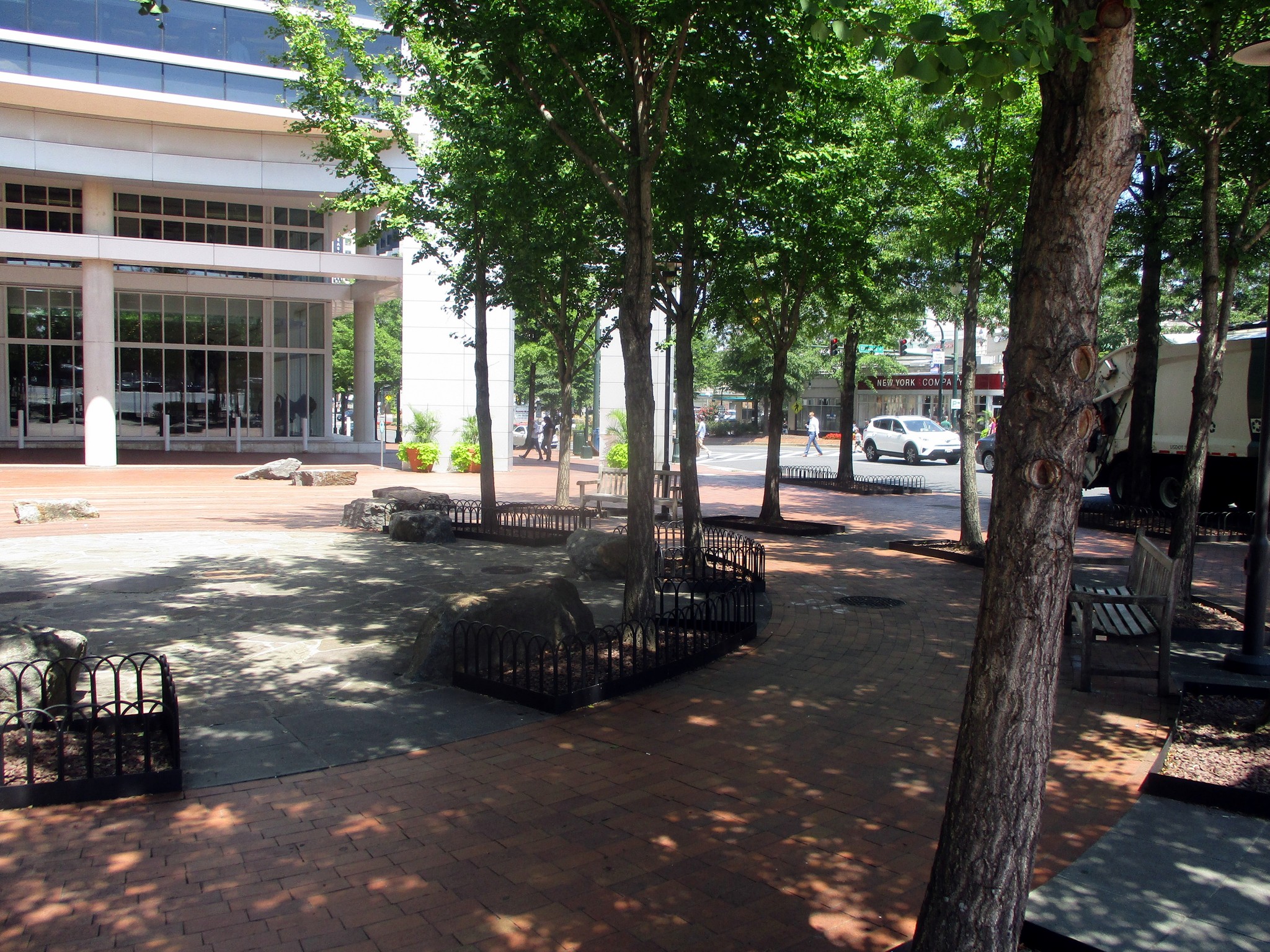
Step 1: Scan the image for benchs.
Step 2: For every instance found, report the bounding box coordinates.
[574,464,685,531]
[1065,527,1180,700]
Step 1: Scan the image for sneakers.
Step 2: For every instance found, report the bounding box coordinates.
[519,455,525,460]
[537,458,544,461]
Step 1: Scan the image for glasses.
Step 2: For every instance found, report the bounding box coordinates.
[808,415,811,416]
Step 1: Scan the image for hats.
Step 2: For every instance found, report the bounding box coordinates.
[528,417,534,421]
[864,418,870,421]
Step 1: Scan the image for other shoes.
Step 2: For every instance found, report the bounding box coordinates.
[708,450,712,458]
[801,454,807,457]
[853,451,855,453]
[857,450,862,453]
[816,453,823,456]
[544,455,551,461]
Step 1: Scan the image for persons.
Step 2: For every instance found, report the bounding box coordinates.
[781,417,789,435]
[851,429,862,454]
[541,416,556,463]
[332,396,387,420]
[518,417,544,462]
[928,409,931,419]
[939,416,952,431]
[987,417,994,436]
[852,421,860,432]
[695,415,712,459]
[909,404,915,415]
[800,411,823,457]
[989,418,997,435]
[864,418,868,421]
[220,392,227,408]
[862,420,869,433]
[942,407,948,416]
[896,404,905,416]
[805,417,810,437]
[885,405,890,415]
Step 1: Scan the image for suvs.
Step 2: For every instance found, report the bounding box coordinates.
[863,415,961,468]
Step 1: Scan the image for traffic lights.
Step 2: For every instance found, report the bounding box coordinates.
[829,337,838,356]
[899,338,907,357]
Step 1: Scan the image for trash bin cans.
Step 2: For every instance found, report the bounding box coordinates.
[572,430,585,455]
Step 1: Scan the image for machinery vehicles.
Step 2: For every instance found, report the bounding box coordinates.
[1082,317,1270,509]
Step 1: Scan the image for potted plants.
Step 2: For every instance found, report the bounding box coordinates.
[450,413,482,472]
[395,402,441,473]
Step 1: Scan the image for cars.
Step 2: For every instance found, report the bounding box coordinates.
[513,425,558,449]
[339,423,354,436]
[337,409,353,421]
[975,433,996,473]
